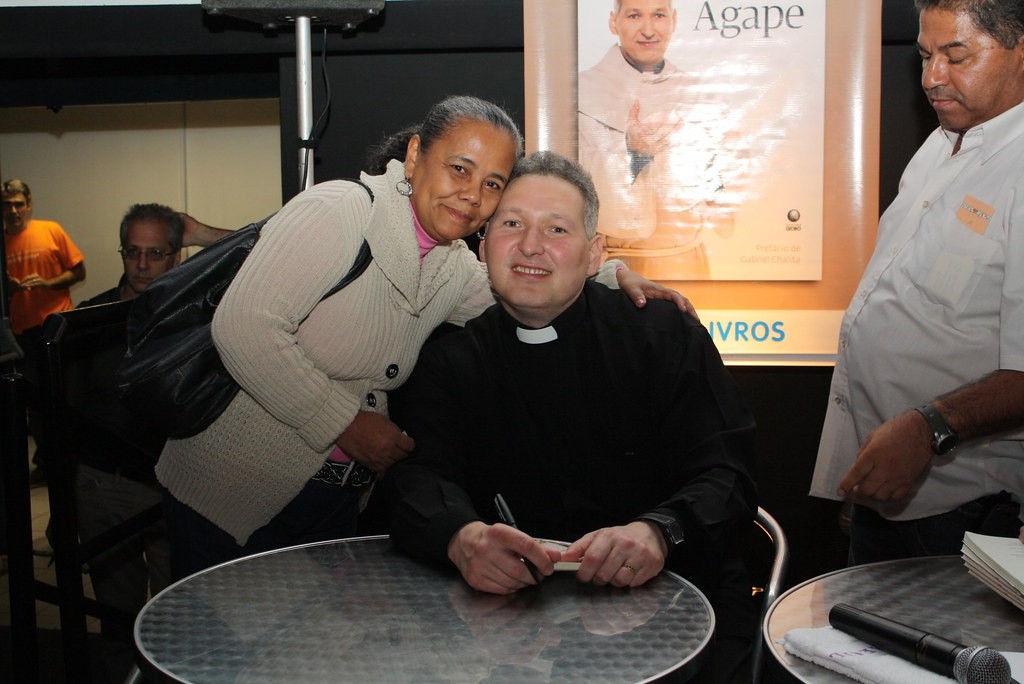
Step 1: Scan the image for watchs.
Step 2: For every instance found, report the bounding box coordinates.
[915,404,960,456]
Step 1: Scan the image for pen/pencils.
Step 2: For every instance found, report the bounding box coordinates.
[494,493,545,584]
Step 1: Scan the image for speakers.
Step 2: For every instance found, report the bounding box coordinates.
[200,1,386,34]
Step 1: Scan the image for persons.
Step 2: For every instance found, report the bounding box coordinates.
[154,95,700,583]
[579,0,718,280]
[71,204,237,639]
[806,0,1024,568]
[0,180,85,484]
[389,155,744,595]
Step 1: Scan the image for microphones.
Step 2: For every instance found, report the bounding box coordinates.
[829,602,1011,684]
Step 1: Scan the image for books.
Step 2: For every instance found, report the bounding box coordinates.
[520,536,581,571]
[961,531,1024,611]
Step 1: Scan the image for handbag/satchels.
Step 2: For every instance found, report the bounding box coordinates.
[117,175,374,440]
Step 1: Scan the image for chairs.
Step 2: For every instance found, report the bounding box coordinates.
[709,509,787,684]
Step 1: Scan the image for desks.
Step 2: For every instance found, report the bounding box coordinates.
[765,555,1024,684]
[134,535,713,684]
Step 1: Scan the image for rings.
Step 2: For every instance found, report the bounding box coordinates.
[624,564,636,573]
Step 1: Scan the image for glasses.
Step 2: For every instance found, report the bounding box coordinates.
[118,245,178,262]
